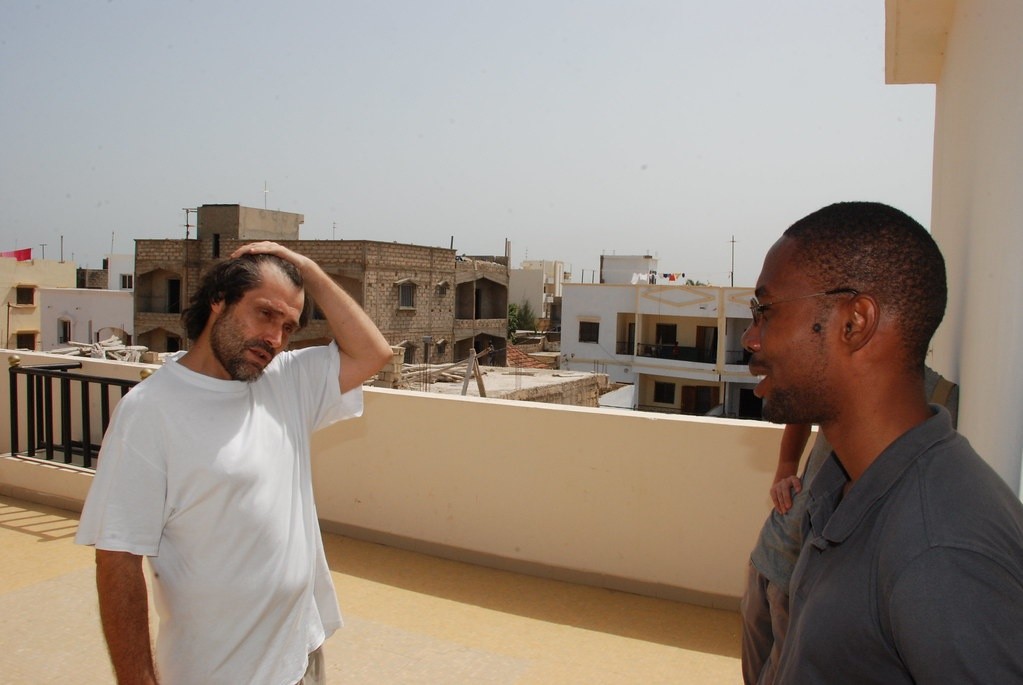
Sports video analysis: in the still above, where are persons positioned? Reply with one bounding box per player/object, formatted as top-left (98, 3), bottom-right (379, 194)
top-left (73, 241), bottom-right (393, 685)
top-left (741, 201), bottom-right (1023, 685)
top-left (770, 364), bottom-right (960, 514)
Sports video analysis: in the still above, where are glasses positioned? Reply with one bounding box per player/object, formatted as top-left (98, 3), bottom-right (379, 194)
top-left (749, 286), bottom-right (859, 327)
